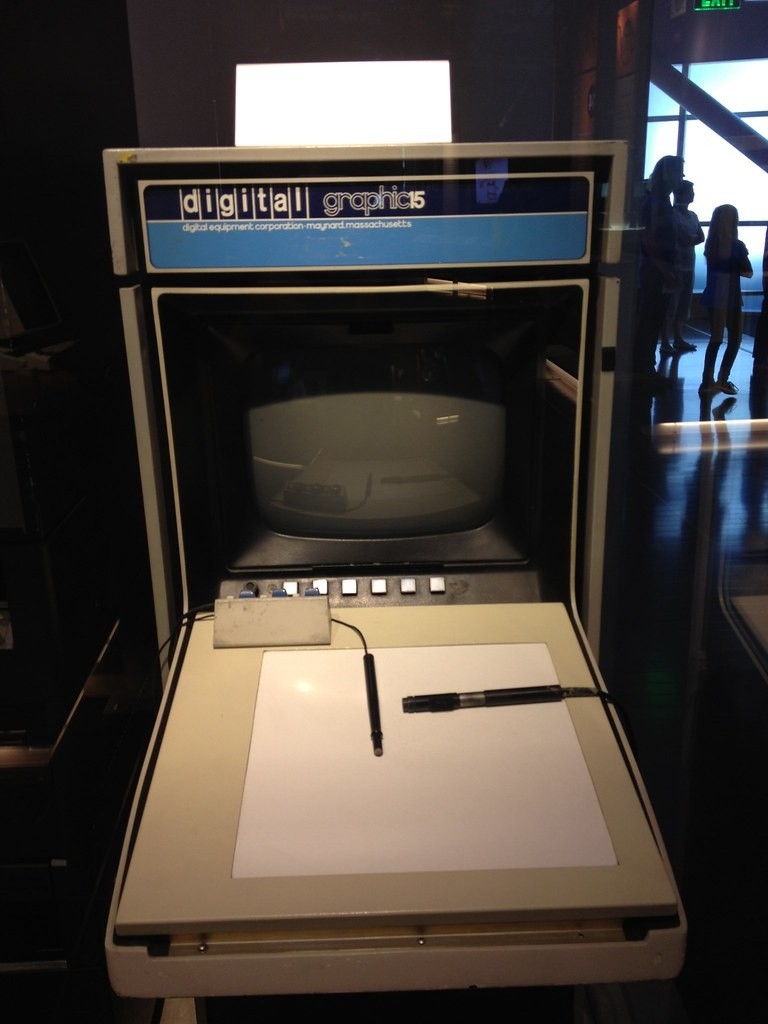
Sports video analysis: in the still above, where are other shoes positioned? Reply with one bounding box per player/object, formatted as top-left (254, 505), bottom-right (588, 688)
top-left (674, 341), bottom-right (696, 349)
top-left (699, 381), bottom-right (721, 394)
top-left (716, 380), bottom-right (737, 394)
top-left (660, 342), bottom-right (679, 353)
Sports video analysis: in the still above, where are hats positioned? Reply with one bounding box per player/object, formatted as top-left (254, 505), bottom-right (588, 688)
top-left (673, 181), bottom-right (691, 195)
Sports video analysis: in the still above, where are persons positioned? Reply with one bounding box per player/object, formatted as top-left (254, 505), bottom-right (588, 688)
top-left (634, 156), bottom-right (704, 386)
top-left (753, 229), bottom-right (768, 370)
top-left (699, 205), bottom-right (752, 394)
